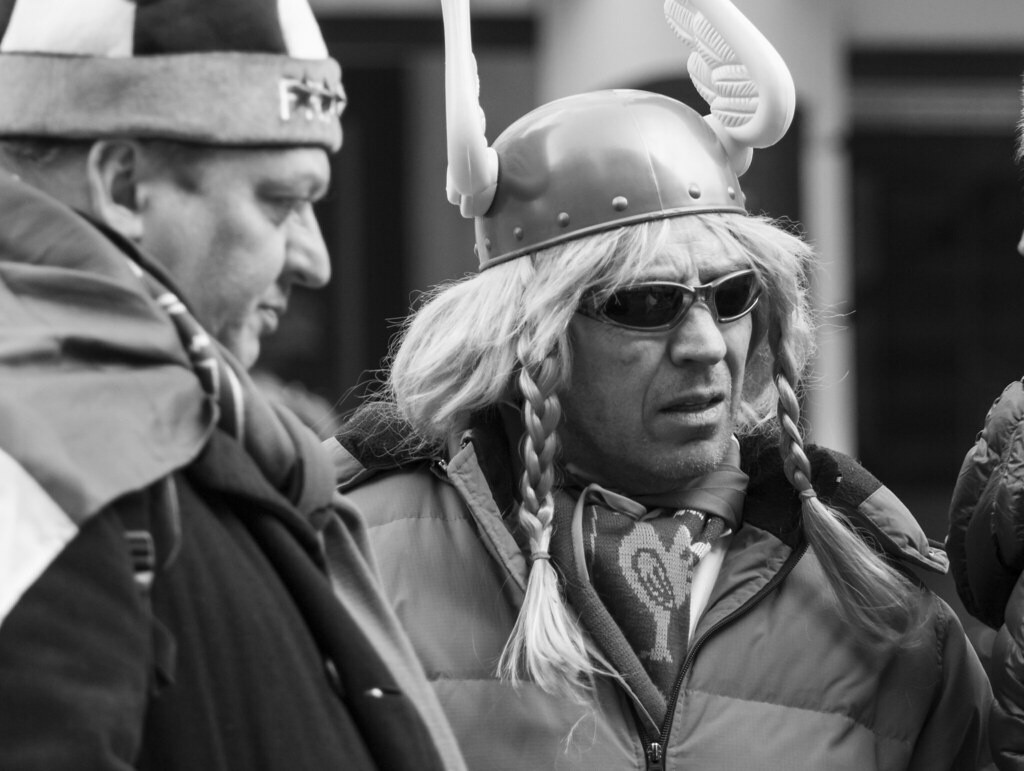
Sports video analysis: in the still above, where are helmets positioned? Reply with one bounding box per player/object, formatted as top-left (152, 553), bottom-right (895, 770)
top-left (440, 0), bottom-right (796, 272)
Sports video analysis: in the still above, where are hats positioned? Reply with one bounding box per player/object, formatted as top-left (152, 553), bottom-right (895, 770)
top-left (0, 0), bottom-right (346, 153)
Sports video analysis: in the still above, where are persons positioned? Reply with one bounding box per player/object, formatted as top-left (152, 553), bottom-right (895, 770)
top-left (0, 0), bottom-right (1024, 771)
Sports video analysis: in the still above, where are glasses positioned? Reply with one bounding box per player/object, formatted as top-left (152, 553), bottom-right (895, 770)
top-left (575, 268), bottom-right (767, 332)
